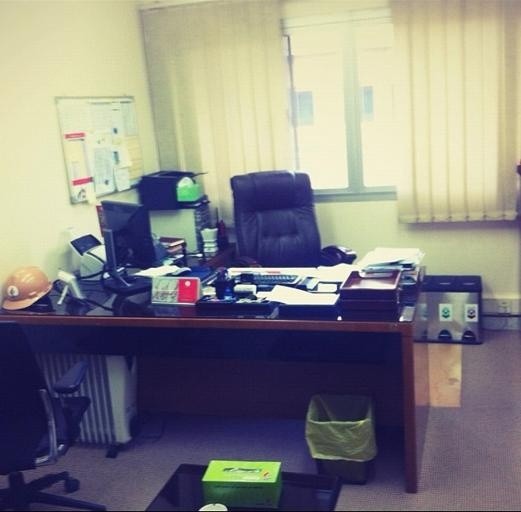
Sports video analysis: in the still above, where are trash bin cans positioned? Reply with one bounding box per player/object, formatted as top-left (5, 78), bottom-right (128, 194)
top-left (307, 393), bottom-right (374, 485)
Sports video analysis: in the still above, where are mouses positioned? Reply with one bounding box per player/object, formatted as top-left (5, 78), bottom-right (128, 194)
top-left (171, 267), bottom-right (192, 276)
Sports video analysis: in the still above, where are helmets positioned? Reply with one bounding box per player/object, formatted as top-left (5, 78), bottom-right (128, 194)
top-left (2, 266), bottom-right (52, 310)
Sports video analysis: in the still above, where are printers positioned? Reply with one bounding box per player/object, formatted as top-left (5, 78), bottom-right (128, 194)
top-left (68, 233), bottom-right (125, 281)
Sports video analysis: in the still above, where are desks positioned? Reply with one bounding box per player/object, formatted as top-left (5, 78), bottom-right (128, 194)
top-left (0, 273), bottom-right (431, 495)
top-left (144, 463), bottom-right (342, 512)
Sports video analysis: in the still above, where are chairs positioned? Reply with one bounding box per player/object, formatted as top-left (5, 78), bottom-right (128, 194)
top-left (230, 170), bottom-right (358, 266)
top-left (0, 320), bottom-right (105, 511)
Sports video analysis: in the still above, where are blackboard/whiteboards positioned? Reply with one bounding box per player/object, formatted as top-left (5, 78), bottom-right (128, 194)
top-left (54, 96), bottom-right (144, 204)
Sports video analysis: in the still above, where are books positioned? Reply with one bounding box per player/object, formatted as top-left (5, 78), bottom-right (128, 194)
top-left (159, 236), bottom-right (186, 248)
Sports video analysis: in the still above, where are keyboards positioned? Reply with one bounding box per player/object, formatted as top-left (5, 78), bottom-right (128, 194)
top-left (228, 273), bottom-right (302, 285)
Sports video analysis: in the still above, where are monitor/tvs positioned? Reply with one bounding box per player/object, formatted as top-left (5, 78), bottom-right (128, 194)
top-left (101, 200), bottom-right (159, 294)
